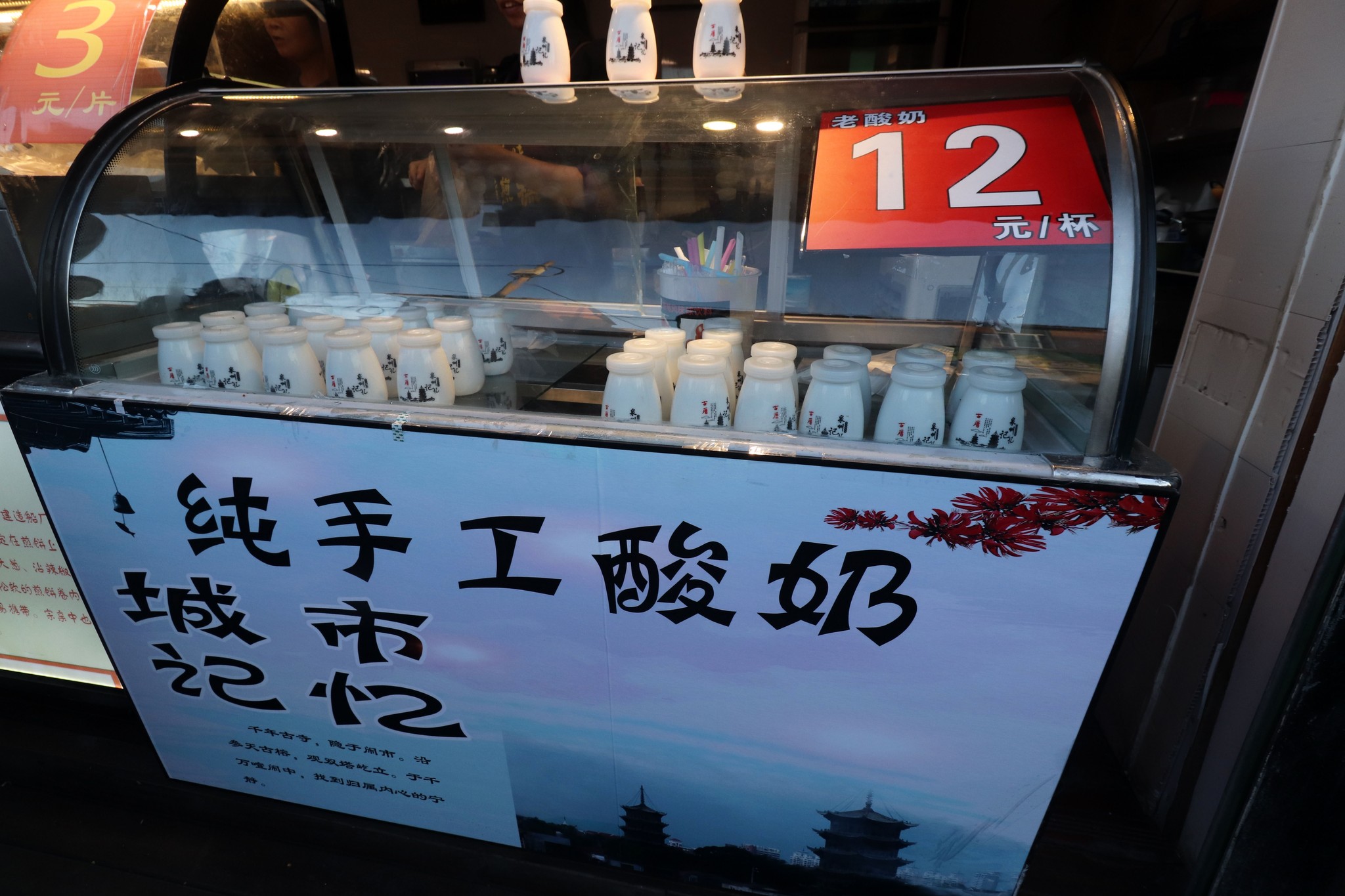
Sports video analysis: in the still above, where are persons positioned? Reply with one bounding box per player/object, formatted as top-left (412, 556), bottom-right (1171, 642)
top-left (128, 0), bottom-right (402, 218)
top-left (408, 0), bottom-right (618, 222)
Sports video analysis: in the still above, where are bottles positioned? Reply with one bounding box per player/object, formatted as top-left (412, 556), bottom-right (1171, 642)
top-left (703, 316), bottom-right (742, 329)
top-left (670, 353), bottom-right (732, 427)
top-left (947, 365), bottom-right (1028, 450)
top-left (798, 359), bottom-right (864, 440)
top-left (151, 292), bottom-right (515, 409)
top-left (873, 362), bottom-right (947, 445)
top-left (733, 355), bottom-right (797, 435)
top-left (750, 341), bottom-right (799, 412)
top-left (945, 349), bottom-right (1017, 441)
top-left (822, 344), bottom-right (872, 432)
top-left (692, 0), bottom-right (746, 100)
top-left (645, 327), bottom-right (687, 391)
top-left (519, 0), bottom-right (575, 102)
top-left (605, 0), bottom-right (660, 103)
top-left (702, 327), bottom-right (746, 406)
top-left (686, 339), bottom-right (736, 421)
top-left (600, 352), bottom-right (663, 426)
top-left (623, 337), bottom-right (674, 422)
top-left (894, 347), bottom-right (947, 368)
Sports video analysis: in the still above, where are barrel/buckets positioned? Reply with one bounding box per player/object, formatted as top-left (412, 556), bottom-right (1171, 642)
top-left (656, 265), bottom-right (762, 358)
